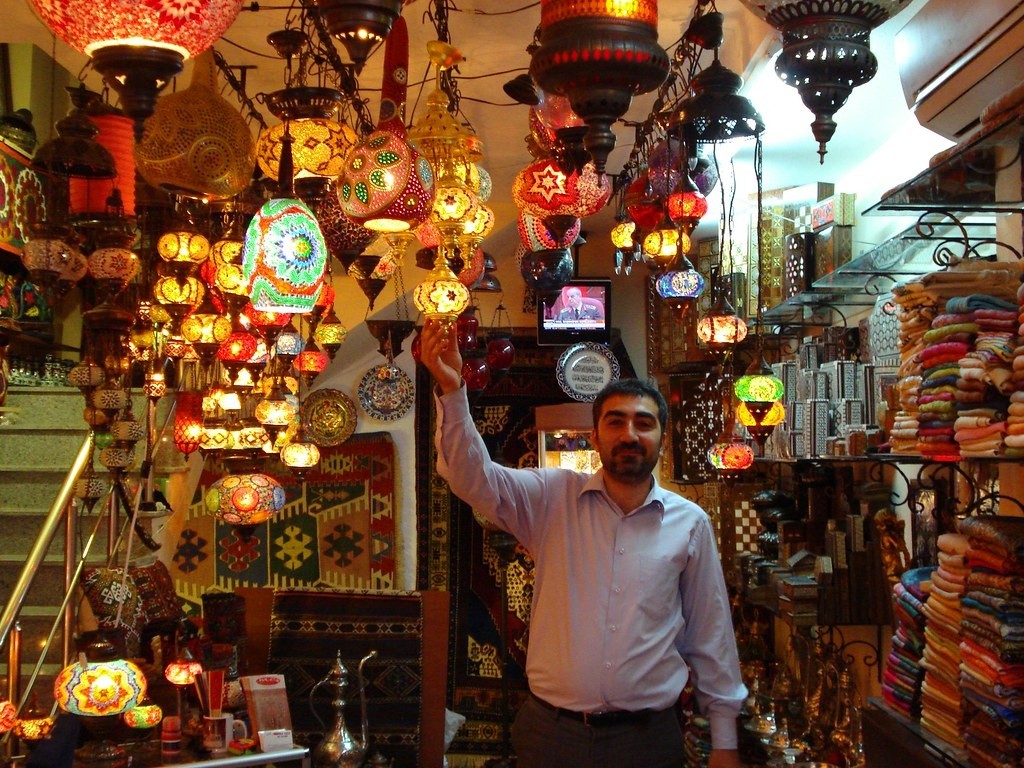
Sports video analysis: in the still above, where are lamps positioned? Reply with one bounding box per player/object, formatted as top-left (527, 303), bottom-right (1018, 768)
top-left (0, 0), bottom-right (912, 743)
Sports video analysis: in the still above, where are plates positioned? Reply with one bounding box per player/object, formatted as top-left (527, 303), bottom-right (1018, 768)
top-left (358, 365), bottom-right (414, 420)
top-left (301, 389), bottom-right (357, 447)
top-left (556, 342), bottom-right (620, 403)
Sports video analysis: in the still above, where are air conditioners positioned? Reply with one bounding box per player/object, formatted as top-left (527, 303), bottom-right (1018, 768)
top-left (893, 0), bottom-right (1024, 145)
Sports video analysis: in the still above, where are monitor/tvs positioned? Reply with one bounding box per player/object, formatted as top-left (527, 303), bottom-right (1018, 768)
top-left (536, 279), bottom-right (612, 347)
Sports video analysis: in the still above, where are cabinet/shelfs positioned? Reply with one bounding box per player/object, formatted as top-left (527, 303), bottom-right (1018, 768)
top-left (747, 201), bottom-right (1024, 768)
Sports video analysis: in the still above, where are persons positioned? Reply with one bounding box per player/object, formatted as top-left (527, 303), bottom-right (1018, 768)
top-left (557, 287), bottom-right (603, 320)
top-left (414, 316), bottom-right (751, 768)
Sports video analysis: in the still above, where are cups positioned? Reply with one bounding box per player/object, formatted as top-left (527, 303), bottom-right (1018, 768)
top-left (203, 713), bottom-right (247, 753)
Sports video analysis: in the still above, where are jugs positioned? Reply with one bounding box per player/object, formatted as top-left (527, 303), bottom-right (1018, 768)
top-left (734, 610), bottom-right (867, 768)
top-left (308, 648), bottom-right (377, 767)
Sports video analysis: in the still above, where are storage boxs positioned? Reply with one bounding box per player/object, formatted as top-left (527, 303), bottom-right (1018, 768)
top-left (771, 344), bottom-right (876, 457)
